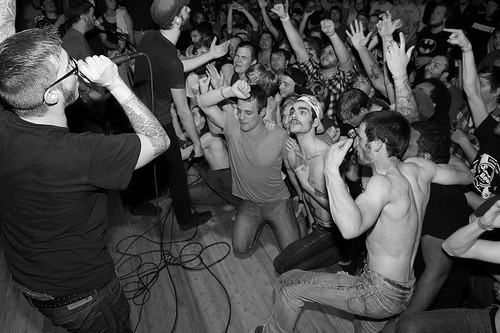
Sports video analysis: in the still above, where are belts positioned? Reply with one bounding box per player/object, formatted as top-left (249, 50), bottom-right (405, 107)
top-left (384, 278), bottom-right (410, 291)
top-left (314, 217), bottom-right (334, 227)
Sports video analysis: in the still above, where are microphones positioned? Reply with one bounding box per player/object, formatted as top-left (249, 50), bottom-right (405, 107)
top-left (80, 53), bottom-right (144, 77)
top-left (94, 20), bottom-right (118, 28)
top-left (189, 150), bottom-right (195, 161)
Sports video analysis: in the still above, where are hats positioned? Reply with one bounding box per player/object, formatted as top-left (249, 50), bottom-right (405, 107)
top-left (150, 0), bottom-right (190, 25)
top-left (69, 1), bottom-right (92, 19)
top-left (297, 94), bottom-right (325, 134)
top-left (283, 68), bottom-right (308, 86)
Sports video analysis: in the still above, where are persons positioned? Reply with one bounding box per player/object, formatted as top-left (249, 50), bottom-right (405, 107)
top-left (0, 0), bottom-right (500, 333)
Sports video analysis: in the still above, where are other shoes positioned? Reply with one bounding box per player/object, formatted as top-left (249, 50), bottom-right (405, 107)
top-left (255, 326), bottom-right (264, 333)
top-left (130, 203), bottom-right (162, 216)
top-left (179, 210), bottom-right (212, 231)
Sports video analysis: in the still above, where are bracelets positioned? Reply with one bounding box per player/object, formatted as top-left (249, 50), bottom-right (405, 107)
top-left (220, 87), bottom-right (227, 99)
top-left (462, 47), bottom-right (473, 53)
top-left (478, 216), bottom-right (491, 231)
top-left (325, 30), bottom-right (335, 36)
top-left (391, 72), bottom-right (408, 80)
top-left (279, 13), bottom-right (290, 22)
top-left (172, 114), bottom-right (178, 117)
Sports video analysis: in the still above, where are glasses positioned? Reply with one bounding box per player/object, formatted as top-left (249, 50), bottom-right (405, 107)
top-left (42, 56), bottom-right (78, 102)
